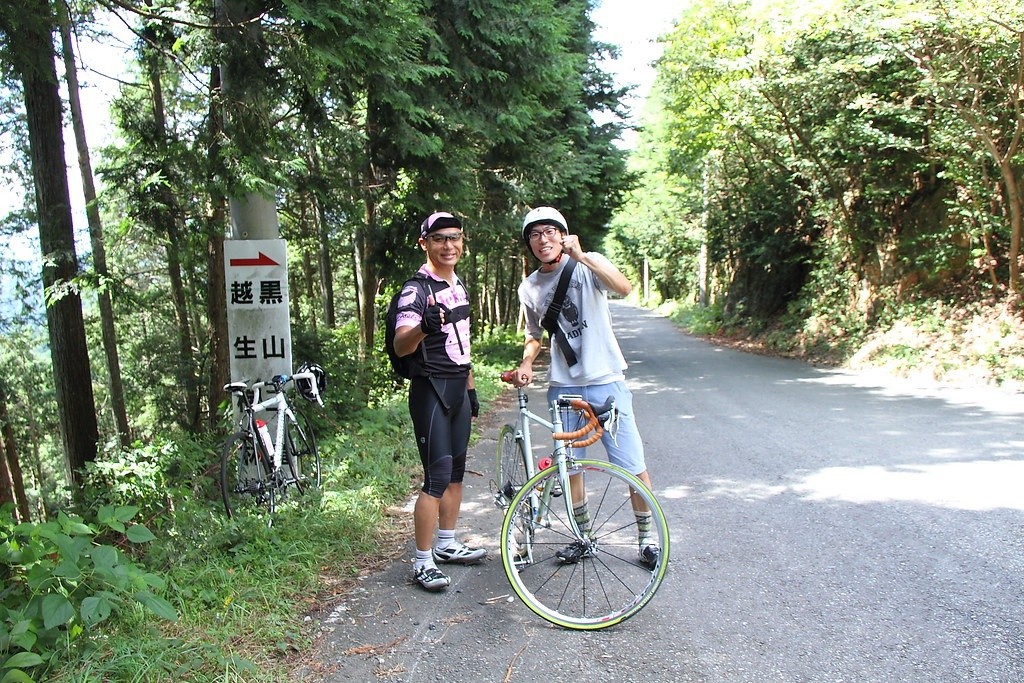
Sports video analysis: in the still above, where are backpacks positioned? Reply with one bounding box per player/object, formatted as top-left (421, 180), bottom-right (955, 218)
top-left (385, 276), bottom-right (437, 380)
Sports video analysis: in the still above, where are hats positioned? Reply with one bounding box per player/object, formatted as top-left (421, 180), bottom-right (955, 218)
top-left (420, 212), bottom-right (462, 237)
top-left (522, 206), bottom-right (569, 239)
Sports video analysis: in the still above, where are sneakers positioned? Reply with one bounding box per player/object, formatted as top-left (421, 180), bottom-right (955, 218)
top-left (638, 544), bottom-right (659, 564)
top-left (556, 537), bottom-right (599, 563)
top-left (413, 560), bottom-right (450, 589)
top-left (432, 541), bottom-right (487, 564)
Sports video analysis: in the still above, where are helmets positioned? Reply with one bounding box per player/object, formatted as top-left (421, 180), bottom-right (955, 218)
top-left (296, 363), bottom-right (326, 402)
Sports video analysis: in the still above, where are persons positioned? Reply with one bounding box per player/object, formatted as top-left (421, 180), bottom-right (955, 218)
top-left (515, 207), bottom-right (672, 571)
top-left (394, 210), bottom-right (490, 587)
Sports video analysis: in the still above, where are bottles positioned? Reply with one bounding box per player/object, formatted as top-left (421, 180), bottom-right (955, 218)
top-left (255, 419), bottom-right (275, 456)
top-left (534, 458), bottom-right (553, 492)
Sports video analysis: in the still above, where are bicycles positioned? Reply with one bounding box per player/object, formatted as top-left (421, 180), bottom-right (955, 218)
top-left (490, 367), bottom-right (671, 632)
top-left (216, 365), bottom-right (323, 530)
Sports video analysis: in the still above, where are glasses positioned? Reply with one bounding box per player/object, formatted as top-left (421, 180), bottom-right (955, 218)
top-left (528, 227), bottom-right (560, 241)
top-left (425, 234), bottom-right (462, 242)
